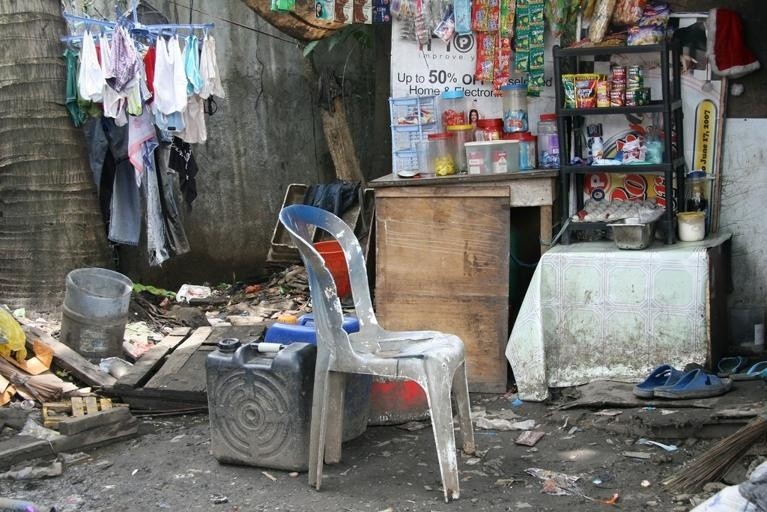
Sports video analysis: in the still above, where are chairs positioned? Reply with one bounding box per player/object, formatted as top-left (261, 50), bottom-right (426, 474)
top-left (278, 205), bottom-right (475, 504)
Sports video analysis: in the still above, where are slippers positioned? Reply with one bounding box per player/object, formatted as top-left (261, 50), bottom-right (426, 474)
top-left (654, 369), bottom-right (733, 398)
top-left (717, 357), bottom-right (747, 377)
top-left (634, 365), bottom-right (686, 398)
top-left (729, 361), bottom-right (767, 381)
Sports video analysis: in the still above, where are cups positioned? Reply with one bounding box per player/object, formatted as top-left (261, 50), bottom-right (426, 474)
top-left (635, 87), bottom-right (651, 106)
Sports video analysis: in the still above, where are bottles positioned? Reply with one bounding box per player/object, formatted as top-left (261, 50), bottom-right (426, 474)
top-left (429, 133), bottom-right (457, 177)
top-left (447, 125), bottom-right (474, 175)
top-left (502, 84), bottom-right (529, 133)
top-left (538, 113), bottom-right (560, 169)
top-left (474, 119), bottom-right (503, 141)
top-left (440, 91), bottom-right (467, 133)
top-left (626, 65), bottom-right (643, 88)
top-left (687, 172), bottom-right (707, 211)
top-left (677, 213), bottom-right (705, 242)
top-left (519, 136), bottom-right (538, 170)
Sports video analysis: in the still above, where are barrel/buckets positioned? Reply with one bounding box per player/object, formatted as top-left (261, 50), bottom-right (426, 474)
top-left (204, 338), bottom-right (317, 473)
top-left (60, 267), bottom-right (135, 365)
top-left (263, 314), bottom-right (369, 442)
top-left (313, 240), bottom-right (349, 297)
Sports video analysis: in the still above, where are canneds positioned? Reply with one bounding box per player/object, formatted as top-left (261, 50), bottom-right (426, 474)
top-left (643, 88), bottom-right (651, 105)
top-left (595, 80), bottom-right (609, 108)
top-left (626, 89), bottom-right (643, 106)
top-left (610, 89), bottom-right (626, 107)
top-left (613, 65), bottom-right (626, 89)
top-left (591, 137), bottom-right (603, 161)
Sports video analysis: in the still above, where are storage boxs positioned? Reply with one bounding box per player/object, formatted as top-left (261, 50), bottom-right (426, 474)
top-left (464, 138), bottom-right (521, 177)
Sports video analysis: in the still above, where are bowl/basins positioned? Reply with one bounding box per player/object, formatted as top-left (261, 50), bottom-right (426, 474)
top-left (606, 218), bottom-right (655, 250)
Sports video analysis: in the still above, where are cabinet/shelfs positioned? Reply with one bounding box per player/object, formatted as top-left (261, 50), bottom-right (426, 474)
top-left (552, 30), bottom-right (685, 246)
top-left (368, 169), bottom-right (563, 394)
top-left (542, 233), bottom-right (734, 386)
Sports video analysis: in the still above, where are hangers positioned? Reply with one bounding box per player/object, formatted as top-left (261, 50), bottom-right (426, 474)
top-left (57, 0), bottom-right (216, 51)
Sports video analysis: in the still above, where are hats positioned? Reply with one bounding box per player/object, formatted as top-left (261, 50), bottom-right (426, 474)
top-left (676, 22), bottom-right (706, 52)
top-left (701, 8), bottom-right (760, 96)
